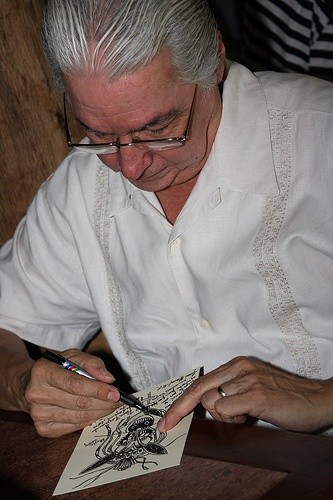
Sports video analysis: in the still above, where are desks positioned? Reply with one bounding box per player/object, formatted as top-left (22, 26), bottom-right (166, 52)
top-left (0, 410), bottom-right (333, 499)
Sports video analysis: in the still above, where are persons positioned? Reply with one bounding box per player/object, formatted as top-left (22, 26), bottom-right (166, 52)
top-left (0, 0), bottom-right (333, 437)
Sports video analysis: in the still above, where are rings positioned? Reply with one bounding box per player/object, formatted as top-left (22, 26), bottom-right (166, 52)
top-left (215, 385), bottom-right (226, 398)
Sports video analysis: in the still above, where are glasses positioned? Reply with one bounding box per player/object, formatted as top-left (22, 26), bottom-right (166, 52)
top-left (61, 82), bottom-right (198, 155)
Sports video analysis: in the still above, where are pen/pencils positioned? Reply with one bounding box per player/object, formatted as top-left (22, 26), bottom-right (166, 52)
top-left (42, 349), bottom-right (149, 413)
